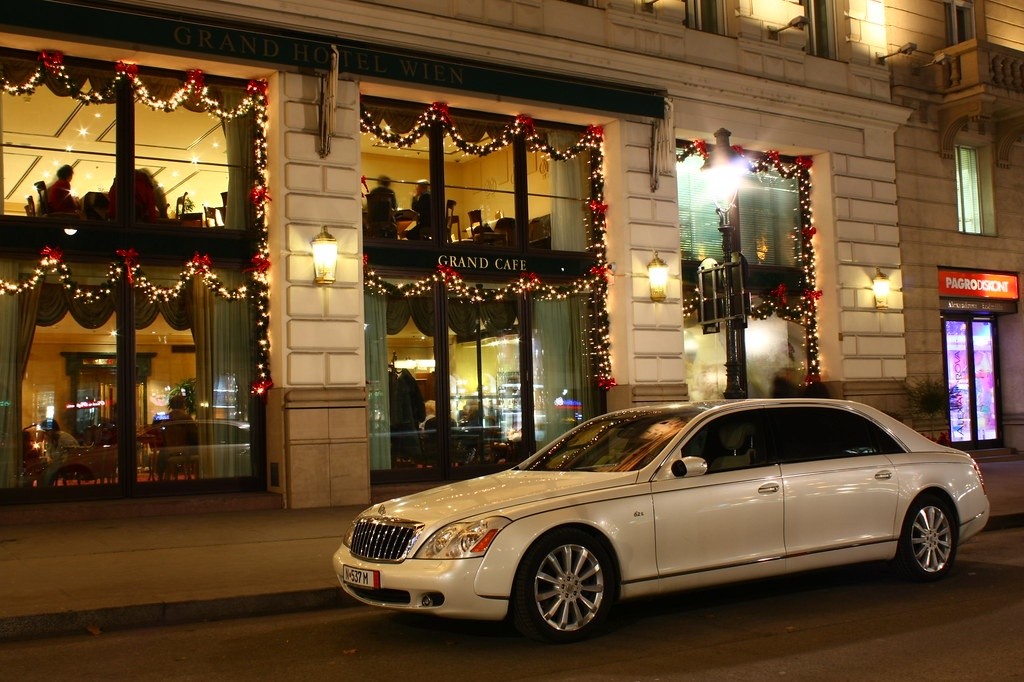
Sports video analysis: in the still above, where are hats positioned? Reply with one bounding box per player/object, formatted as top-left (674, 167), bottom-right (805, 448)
top-left (771, 356), bottom-right (795, 369)
top-left (415, 178), bottom-right (429, 185)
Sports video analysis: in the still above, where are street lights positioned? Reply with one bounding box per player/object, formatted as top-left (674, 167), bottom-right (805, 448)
top-left (699, 128), bottom-right (749, 397)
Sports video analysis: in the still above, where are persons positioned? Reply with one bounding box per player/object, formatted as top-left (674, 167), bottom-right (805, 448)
top-left (368, 176), bottom-right (398, 240)
top-left (407, 179), bottom-right (431, 240)
top-left (47, 165), bottom-right (86, 219)
top-left (169, 396), bottom-right (193, 419)
top-left (40, 419), bottom-right (79, 488)
top-left (140, 169), bottom-right (167, 218)
top-left (420, 400), bottom-right (504, 429)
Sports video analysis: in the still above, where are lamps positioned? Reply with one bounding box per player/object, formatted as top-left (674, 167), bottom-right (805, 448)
top-left (766, 16), bottom-right (808, 42)
top-left (646, 250), bottom-right (669, 301)
top-left (871, 268), bottom-right (891, 311)
top-left (311, 226), bottom-right (336, 287)
top-left (875, 41), bottom-right (917, 65)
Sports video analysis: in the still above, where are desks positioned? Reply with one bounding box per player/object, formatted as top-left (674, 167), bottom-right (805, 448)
top-left (363, 208), bottom-right (419, 239)
top-left (461, 217), bottom-right (540, 245)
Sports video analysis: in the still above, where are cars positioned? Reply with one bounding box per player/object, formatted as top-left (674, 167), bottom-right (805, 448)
top-left (331, 398), bottom-right (990, 643)
top-left (40, 420), bottom-right (254, 488)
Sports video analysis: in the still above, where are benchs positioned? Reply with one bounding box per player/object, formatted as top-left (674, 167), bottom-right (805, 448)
top-left (529, 213), bottom-right (550, 247)
top-left (370, 426), bottom-right (501, 468)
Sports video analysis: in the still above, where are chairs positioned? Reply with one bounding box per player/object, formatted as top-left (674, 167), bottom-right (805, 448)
top-left (175, 192), bottom-right (188, 219)
top-left (711, 420), bottom-right (760, 468)
top-left (203, 204), bottom-right (219, 227)
top-left (447, 215), bottom-right (473, 243)
top-left (468, 209), bottom-right (505, 246)
top-left (421, 200), bottom-right (457, 241)
top-left (364, 193), bottom-right (398, 240)
top-left (24, 179), bottom-right (77, 219)
